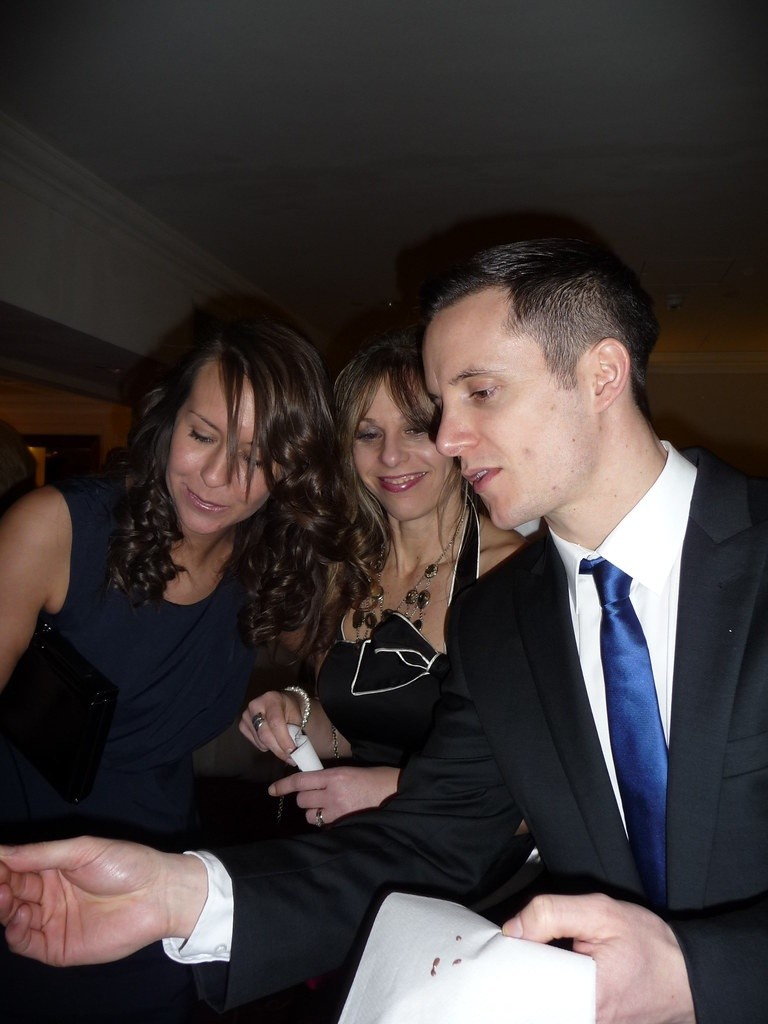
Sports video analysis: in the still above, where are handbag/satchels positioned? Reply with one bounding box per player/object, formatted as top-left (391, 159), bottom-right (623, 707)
top-left (1, 607), bottom-right (122, 808)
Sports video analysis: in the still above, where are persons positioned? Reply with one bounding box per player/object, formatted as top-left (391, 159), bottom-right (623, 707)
top-left (238, 328), bottom-right (536, 1023)
top-left (0, 209), bottom-right (768, 1024)
top-left (0, 292), bottom-right (353, 1024)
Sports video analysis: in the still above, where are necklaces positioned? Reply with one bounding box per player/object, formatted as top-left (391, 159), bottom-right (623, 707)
top-left (347, 502), bottom-right (466, 641)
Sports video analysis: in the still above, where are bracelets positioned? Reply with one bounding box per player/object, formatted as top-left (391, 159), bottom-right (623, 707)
top-left (282, 687), bottom-right (312, 729)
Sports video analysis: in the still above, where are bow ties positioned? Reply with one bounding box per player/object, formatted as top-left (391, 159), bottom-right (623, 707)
top-left (584, 550), bottom-right (671, 905)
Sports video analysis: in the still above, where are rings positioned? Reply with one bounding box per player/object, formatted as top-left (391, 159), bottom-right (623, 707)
top-left (249, 713), bottom-right (265, 731)
top-left (315, 807), bottom-right (325, 828)
